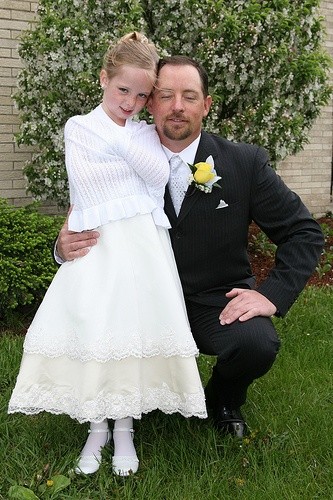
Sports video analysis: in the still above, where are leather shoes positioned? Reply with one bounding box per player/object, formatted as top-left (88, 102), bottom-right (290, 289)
top-left (208, 405), bottom-right (250, 438)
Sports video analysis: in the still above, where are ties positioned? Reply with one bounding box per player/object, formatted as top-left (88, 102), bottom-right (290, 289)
top-left (168, 155), bottom-right (187, 217)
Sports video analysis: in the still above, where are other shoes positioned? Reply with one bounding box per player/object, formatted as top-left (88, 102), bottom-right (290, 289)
top-left (112, 428), bottom-right (140, 476)
top-left (75, 429), bottom-right (111, 474)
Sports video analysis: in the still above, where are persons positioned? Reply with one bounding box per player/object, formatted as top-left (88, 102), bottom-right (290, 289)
top-left (8, 31), bottom-right (208, 477)
top-left (50, 56), bottom-right (325, 449)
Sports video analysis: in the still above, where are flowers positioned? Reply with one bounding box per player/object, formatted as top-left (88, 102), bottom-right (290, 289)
top-left (188, 155), bottom-right (222, 194)
